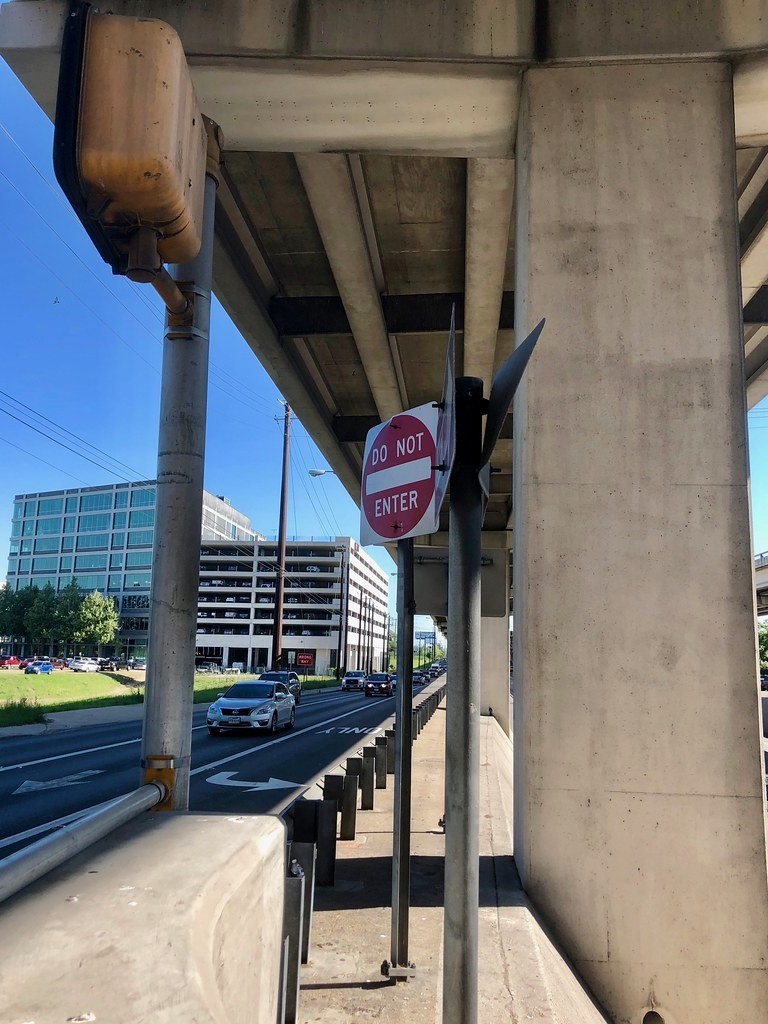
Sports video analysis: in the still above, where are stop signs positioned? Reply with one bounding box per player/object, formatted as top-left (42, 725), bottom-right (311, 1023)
top-left (360, 400), bottom-right (438, 546)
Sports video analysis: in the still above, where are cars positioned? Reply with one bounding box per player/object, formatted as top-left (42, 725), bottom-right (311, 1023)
top-left (257, 629), bottom-right (268, 635)
top-left (307, 565), bottom-right (320, 572)
top-left (225, 612), bottom-right (318, 619)
top-left (203, 549), bottom-right (209, 555)
top-left (286, 630), bottom-right (298, 636)
top-left (198, 612), bottom-right (207, 617)
top-left (224, 629), bottom-right (232, 634)
top-left (228, 566), bottom-right (236, 570)
top-left (240, 628), bottom-right (249, 634)
top-left (207, 680), bottom-right (296, 737)
top-left (197, 628), bottom-right (207, 634)
top-left (200, 579), bottom-right (316, 587)
top-left (391, 659), bottom-right (447, 689)
top-left (302, 630), bottom-right (314, 636)
top-left (198, 596), bottom-right (298, 603)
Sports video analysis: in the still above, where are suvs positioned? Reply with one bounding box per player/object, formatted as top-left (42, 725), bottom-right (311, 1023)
top-left (197, 662), bottom-right (226, 674)
top-left (365, 674), bottom-right (393, 697)
top-left (0, 656), bottom-right (146, 675)
top-left (258, 669), bottom-right (302, 704)
top-left (342, 670), bottom-right (368, 691)
